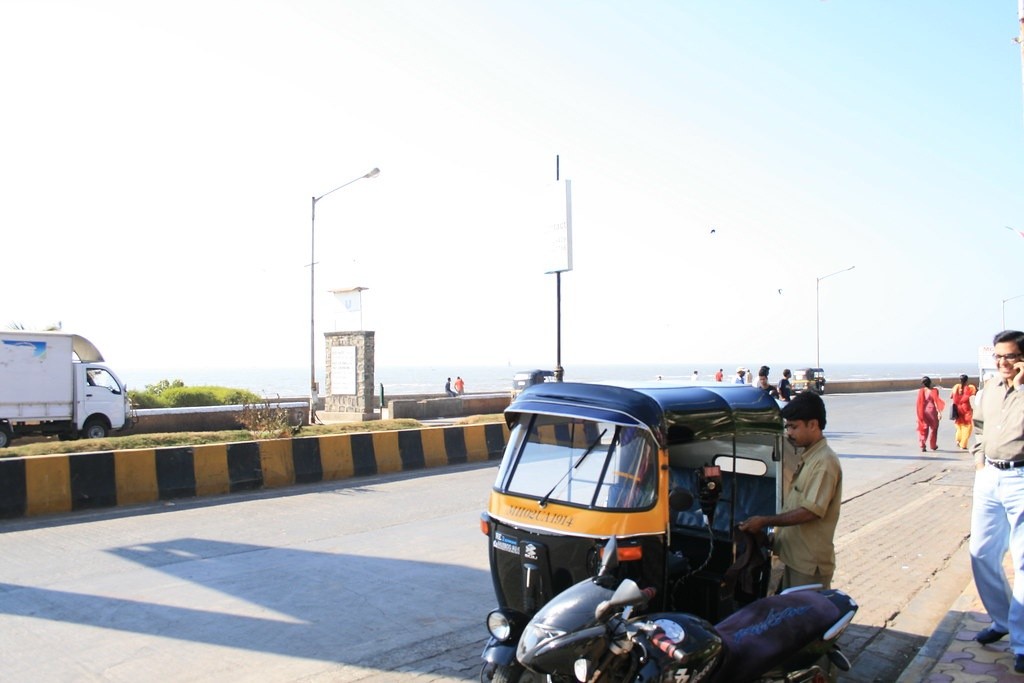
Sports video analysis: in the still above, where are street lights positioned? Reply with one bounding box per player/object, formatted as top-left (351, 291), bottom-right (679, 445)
top-left (310, 167), bottom-right (381, 424)
top-left (816, 266), bottom-right (856, 377)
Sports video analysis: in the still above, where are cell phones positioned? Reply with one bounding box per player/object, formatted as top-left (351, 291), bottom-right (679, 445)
top-left (1016, 359), bottom-right (1024, 374)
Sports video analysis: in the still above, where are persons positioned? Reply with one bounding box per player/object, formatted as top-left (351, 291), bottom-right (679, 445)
top-left (970, 330), bottom-right (1024, 673)
top-left (917, 376), bottom-right (938, 452)
top-left (735, 368), bottom-right (753, 384)
top-left (454, 377), bottom-right (464, 395)
top-left (691, 371), bottom-right (699, 381)
top-left (445, 378), bottom-right (455, 398)
top-left (739, 391), bottom-right (842, 591)
top-left (586, 425), bottom-right (709, 614)
top-left (657, 376), bottom-right (661, 381)
top-left (752, 366), bottom-right (779, 400)
top-left (777, 369), bottom-right (791, 401)
top-left (950, 375), bottom-right (972, 450)
top-left (716, 368), bottom-right (723, 382)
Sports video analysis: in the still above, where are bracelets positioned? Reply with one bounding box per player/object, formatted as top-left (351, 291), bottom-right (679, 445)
top-left (1014, 384), bottom-right (1024, 392)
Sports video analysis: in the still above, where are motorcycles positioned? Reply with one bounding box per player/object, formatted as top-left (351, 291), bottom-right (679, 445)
top-left (515, 533), bottom-right (858, 683)
top-left (792, 368), bottom-right (826, 396)
top-left (480, 382), bottom-right (809, 683)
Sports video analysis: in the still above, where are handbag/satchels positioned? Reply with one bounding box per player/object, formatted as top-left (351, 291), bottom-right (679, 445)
top-left (948, 403), bottom-right (958, 420)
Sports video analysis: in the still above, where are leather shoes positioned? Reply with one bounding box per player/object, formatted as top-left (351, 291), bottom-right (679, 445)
top-left (1013, 654), bottom-right (1024, 673)
top-left (976, 627), bottom-right (1008, 644)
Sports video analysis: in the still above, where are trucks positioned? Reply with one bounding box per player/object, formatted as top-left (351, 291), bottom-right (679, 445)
top-left (0, 331), bottom-right (142, 448)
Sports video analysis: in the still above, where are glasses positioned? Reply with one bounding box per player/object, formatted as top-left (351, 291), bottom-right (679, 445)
top-left (992, 353), bottom-right (1023, 359)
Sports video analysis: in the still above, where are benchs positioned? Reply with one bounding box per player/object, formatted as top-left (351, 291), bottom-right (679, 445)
top-left (607, 464), bottom-right (776, 542)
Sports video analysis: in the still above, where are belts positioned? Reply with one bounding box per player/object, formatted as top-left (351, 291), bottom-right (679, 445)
top-left (986, 459), bottom-right (1024, 471)
